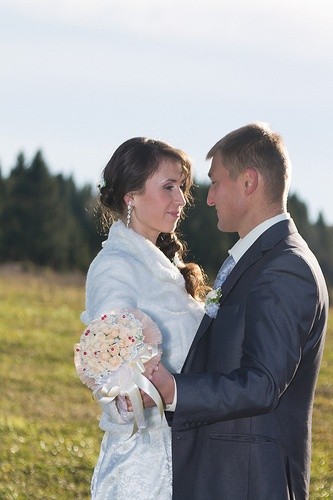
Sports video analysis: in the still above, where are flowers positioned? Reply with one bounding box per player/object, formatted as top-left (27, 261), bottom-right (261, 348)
top-left (204, 287), bottom-right (223, 319)
top-left (72, 304), bottom-right (163, 443)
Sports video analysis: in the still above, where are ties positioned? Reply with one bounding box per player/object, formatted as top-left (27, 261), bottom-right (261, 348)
top-left (213, 252), bottom-right (235, 294)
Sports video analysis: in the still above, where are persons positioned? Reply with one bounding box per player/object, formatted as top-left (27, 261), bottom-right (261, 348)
top-left (124, 125), bottom-right (329, 500)
top-left (80, 136), bottom-right (213, 500)
